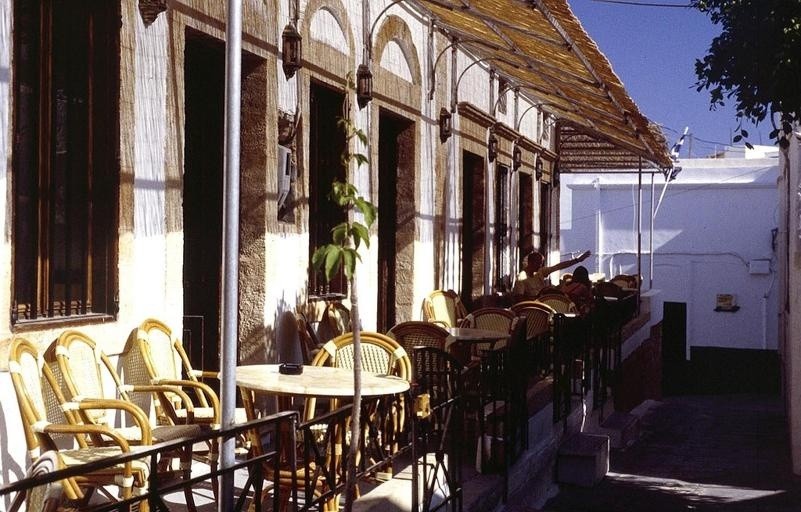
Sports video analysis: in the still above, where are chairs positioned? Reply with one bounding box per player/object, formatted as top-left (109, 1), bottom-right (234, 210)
top-left (9, 337), bottom-right (163, 512)
top-left (462, 306), bottom-right (516, 385)
top-left (385, 321), bottom-right (460, 421)
top-left (609, 274), bottom-right (637, 298)
top-left (592, 282), bottom-right (627, 320)
top-left (422, 290), bottom-right (464, 337)
top-left (135, 317), bottom-right (265, 511)
top-left (301, 331), bottom-right (412, 486)
top-left (534, 295), bottom-right (577, 325)
top-left (510, 302), bottom-right (562, 341)
top-left (55, 329), bottom-right (211, 510)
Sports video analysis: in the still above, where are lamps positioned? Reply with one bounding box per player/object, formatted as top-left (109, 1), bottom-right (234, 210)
top-left (552, 161), bottom-right (560, 187)
top-left (488, 132), bottom-right (499, 162)
top-left (439, 107), bottom-right (453, 143)
top-left (535, 155), bottom-right (544, 181)
top-left (281, 21), bottom-right (303, 80)
top-left (356, 63), bottom-right (373, 109)
top-left (512, 144), bottom-right (522, 172)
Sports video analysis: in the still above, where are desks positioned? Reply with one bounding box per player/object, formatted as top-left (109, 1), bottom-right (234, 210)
top-left (217, 363), bottom-right (409, 510)
top-left (438, 326), bottom-right (512, 417)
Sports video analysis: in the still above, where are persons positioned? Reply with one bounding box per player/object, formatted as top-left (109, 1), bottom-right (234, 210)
top-left (562, 267), bottom-right (591, 295)
top-left (514, 250), bottom-right (591, 297)
